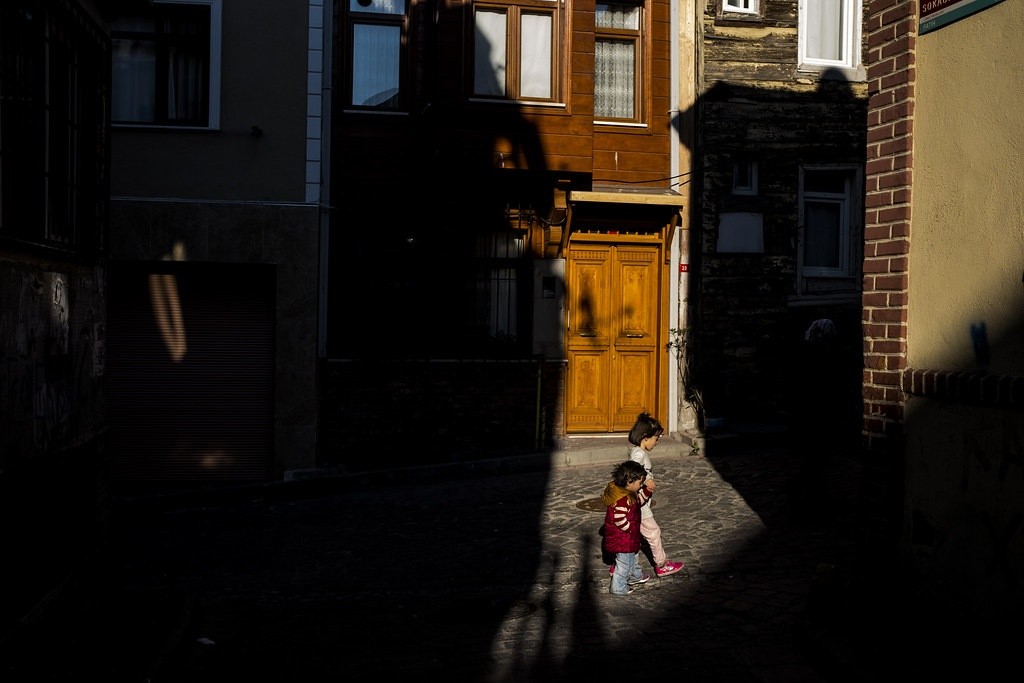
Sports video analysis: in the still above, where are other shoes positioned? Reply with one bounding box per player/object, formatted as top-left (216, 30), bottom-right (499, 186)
top-left (627, 573), bottom-right (651, 585)
top-left (609, 587), bottom-right (634, 595)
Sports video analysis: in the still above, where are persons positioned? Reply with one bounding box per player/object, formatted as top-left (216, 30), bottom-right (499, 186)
top-left (609, 413), bottom-right (683, 577)
top-left (602, 461), bottom-right (655, 595)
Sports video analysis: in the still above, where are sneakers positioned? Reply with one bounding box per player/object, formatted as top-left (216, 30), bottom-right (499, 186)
top-left (655, 560), bottom-right (685, 577)
top-left (609, 564), bottom-right (615, 576)
